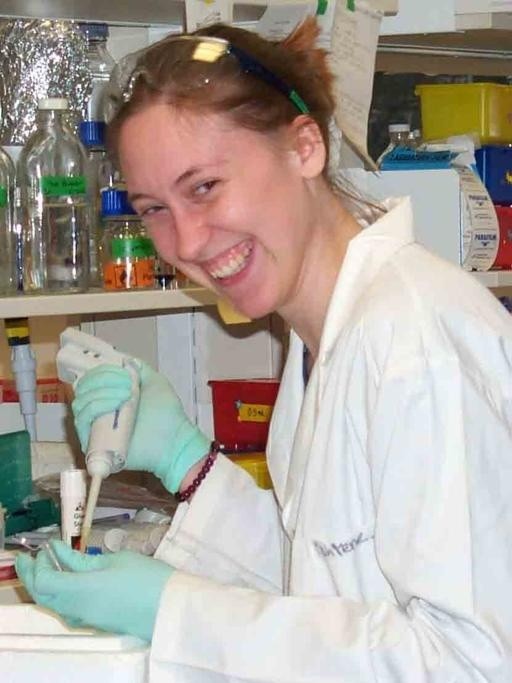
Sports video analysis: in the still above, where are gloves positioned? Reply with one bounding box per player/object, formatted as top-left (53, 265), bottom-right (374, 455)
top-left (71, 361), bottom-right (212, 495)
top-left (15, 539), bottom-right (176, 642)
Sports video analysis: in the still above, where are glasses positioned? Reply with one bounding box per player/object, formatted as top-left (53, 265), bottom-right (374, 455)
top-left (103, 35), bottom-right (311, 125)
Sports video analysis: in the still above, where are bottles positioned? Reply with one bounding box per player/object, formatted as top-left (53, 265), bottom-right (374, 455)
top-left (58, 467), bottom-right (88, 549)
top-left (377, 124), bottom-right (411, 164)
top-left (0, 22), bottom-right (176, 294)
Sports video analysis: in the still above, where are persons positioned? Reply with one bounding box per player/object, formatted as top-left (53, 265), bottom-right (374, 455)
top-left (13, 12), bottom-right (512, 683)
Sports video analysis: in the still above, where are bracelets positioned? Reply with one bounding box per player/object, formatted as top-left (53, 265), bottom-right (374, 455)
top-left (176, 439), bottom-right (220, 501)
